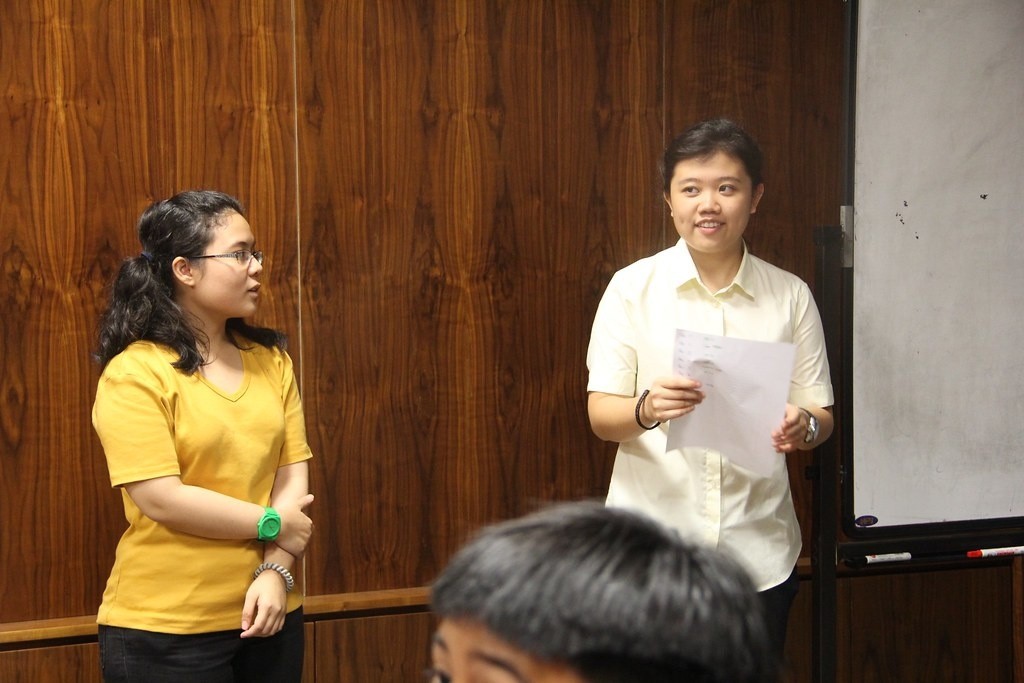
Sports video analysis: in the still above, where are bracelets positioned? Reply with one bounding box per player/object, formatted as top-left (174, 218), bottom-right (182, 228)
top-left (635, 389), bottom-right (661, 430)
top-left (253, 562), bottom-right (295, 592)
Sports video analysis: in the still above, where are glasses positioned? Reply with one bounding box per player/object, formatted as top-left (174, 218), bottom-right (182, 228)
top-left (181, 250), bottom-right (263, 266)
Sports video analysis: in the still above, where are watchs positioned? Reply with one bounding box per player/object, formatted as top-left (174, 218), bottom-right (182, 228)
top-left (256, 507), bottom-right (282, 544)
top-left (801, 408), bottom-right (820, 444)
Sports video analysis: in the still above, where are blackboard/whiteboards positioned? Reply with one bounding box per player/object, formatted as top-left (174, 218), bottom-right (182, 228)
top-left (844, 0), bottom-right (1024, 538)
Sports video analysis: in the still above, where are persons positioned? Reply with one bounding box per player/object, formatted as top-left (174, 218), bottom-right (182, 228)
top-left (91, 191), bottom-right (314, 683)
top-left (421, 501), bottom-right (774, 683)
top-left (584, 117), bottom-right (834, 660)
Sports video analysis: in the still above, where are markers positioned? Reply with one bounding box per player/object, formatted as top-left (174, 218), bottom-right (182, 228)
top-left (845, 552), bottom-right (911, 567)
top-left (967, 545), bottom-right (1024, 558)
